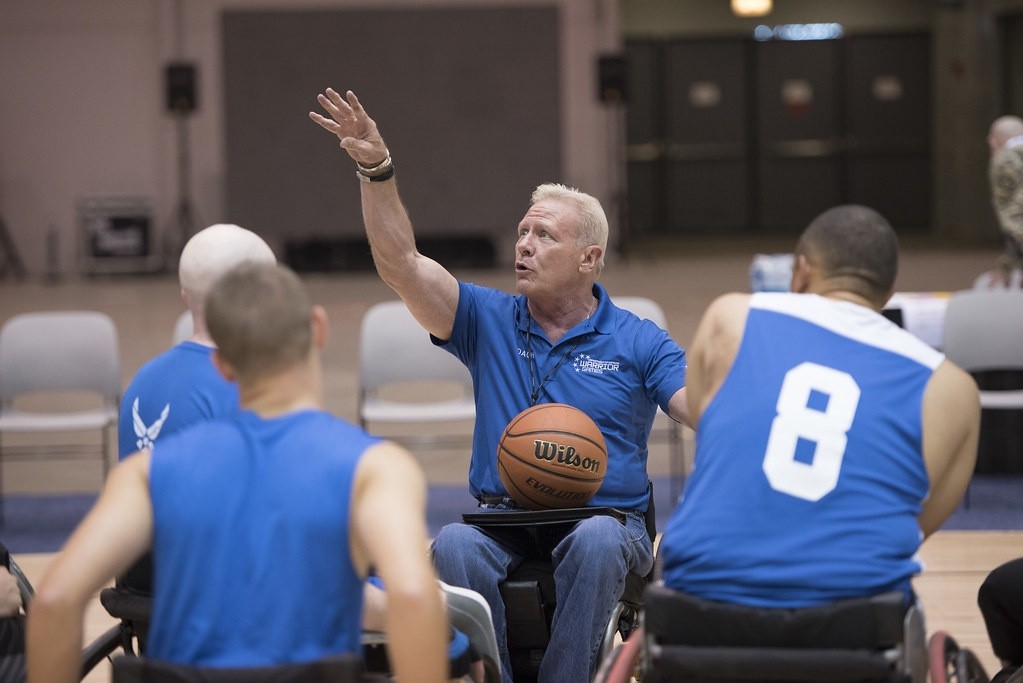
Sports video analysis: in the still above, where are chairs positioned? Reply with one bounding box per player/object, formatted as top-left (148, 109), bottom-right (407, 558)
top-left (607, 296), bottom-right (682, 501)
top-left (1, 312), bottom-right (124, 478)
top-left (940, 290), bottom-right (1023, 501)
top-left (355, 301), bottom-right (475, 435)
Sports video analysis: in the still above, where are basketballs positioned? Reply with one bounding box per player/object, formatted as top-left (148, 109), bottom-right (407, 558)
top-left (496, 401), bottom-right (609, 511)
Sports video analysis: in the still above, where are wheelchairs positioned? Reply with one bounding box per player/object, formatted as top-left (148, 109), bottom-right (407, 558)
top-left (64, 529), bottom-right (990, 683)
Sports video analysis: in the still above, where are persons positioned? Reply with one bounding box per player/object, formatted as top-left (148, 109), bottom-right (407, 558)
top-left (119, 225), bottom-right (485, 682)
top-left (977, 557), bottom-right (1023, 667)
top-left (974, 117), bottom-right (1022, 289)
top-left (0, 565), bottom-right (27, 683)
top-left (661, 207), bottom-right (980, 669)
top-left (310, 87), bottom-right (695, 683)
top-left (27, 261), bottom-right (448, 683)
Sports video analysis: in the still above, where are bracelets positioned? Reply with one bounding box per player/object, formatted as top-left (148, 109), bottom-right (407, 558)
top-left (356, 149), bottom-right (395, 182)
